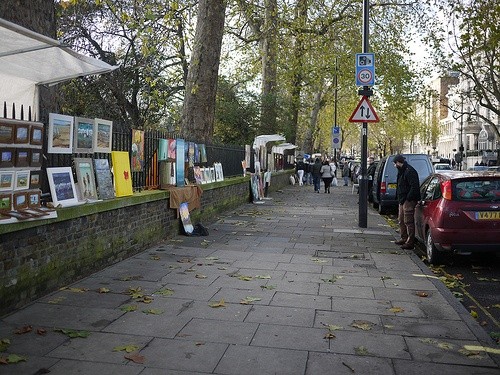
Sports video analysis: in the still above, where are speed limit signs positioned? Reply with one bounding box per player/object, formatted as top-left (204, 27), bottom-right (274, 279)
top-left (358, 69), bottom-right (372, 84)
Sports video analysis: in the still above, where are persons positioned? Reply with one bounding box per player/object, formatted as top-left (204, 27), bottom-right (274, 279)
top-left (393, 152), bottom-right (421, 250)
top-left (311, 157), bottom-right (322, 192)
top-left (451, 158), bottom-right (455, 168)
top-left (320, 160), bottom-right (333, 193)
top-left (294, 156), bottom-right (338, 186)
top-left (474, 159), bottom-right (494, 166)
top-left (366, 156), bottom-right (376, 204)
top-left (342, 161), bottom-right (349, 185)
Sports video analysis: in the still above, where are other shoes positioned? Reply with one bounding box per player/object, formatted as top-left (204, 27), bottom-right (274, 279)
top-left (343, 184), bottom-right (347, 186)
top-left (400, 244), bottom-right (414, 249)
top-left (318, 189), bottom-right (319, 193)
top-left (395, 239), bottom-right (407, 244)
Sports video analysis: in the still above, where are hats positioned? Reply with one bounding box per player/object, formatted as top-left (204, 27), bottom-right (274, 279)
top-left (316, 158), bottom-right (319, 161)
top-left (391, 154), bottom-right (405, 162)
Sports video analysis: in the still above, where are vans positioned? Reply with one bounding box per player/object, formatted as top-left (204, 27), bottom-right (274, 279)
top-left (371, 153), bottom-right (434, 215)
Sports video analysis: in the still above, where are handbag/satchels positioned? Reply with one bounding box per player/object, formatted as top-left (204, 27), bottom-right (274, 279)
top-left (331, 177), bottom-right (337, 185)
top-left (330, 166), bottom-right (335, 176)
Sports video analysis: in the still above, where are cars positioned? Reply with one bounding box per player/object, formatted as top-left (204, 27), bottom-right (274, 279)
top-left (412, 169), bottom-right (500, 265)
top-left (339, 156), bottom-right (378, 203)
top-left (434, 163), bottom-right (452, 175)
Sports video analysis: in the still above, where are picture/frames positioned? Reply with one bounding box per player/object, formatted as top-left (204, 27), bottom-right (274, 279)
top-left (47, 113), bottom-right (73, 154)
top-left (200, 168), bottom-right (216, 184)
top-left (28, 191), bottom-right (41, 207)
top-left (46, 167), bottom-right (78, 208)
top-left (0, 171), bottom-right (15, 191)
top-left (14, 170), bottom-right (30, 190)
top-left (0, 147), bottom-right (16, 168)
top-left (94, 118), bottom-right (113, 153)
top-left (0, 210), bottom-right (34, 219)
top-left (0, 122), bottom-right (15, 144)
top-left (31, 125), bottom-right (44, 145)
top-left (29, 171), bottom-right (43, 189)
top-left (0, 194), bottom-right (12, 219)
top-left (14, 124), bottom-right (30, 144)
top-left (30, 148), bottom-right (43, 167)
top-left (15, 148), bottom-right (31, 167)
top-left (73, 117), bottom-right (95, 153)
top-left (17, 208), bottom-right (50, 217)
top-left (74, 158), bottom-right (99, 201)
top-left (13, 192), bottom-right (28, 210)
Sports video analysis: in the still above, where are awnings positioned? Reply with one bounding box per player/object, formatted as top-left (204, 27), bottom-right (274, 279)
top-left (0, 17), bottom-right (120, 123)
